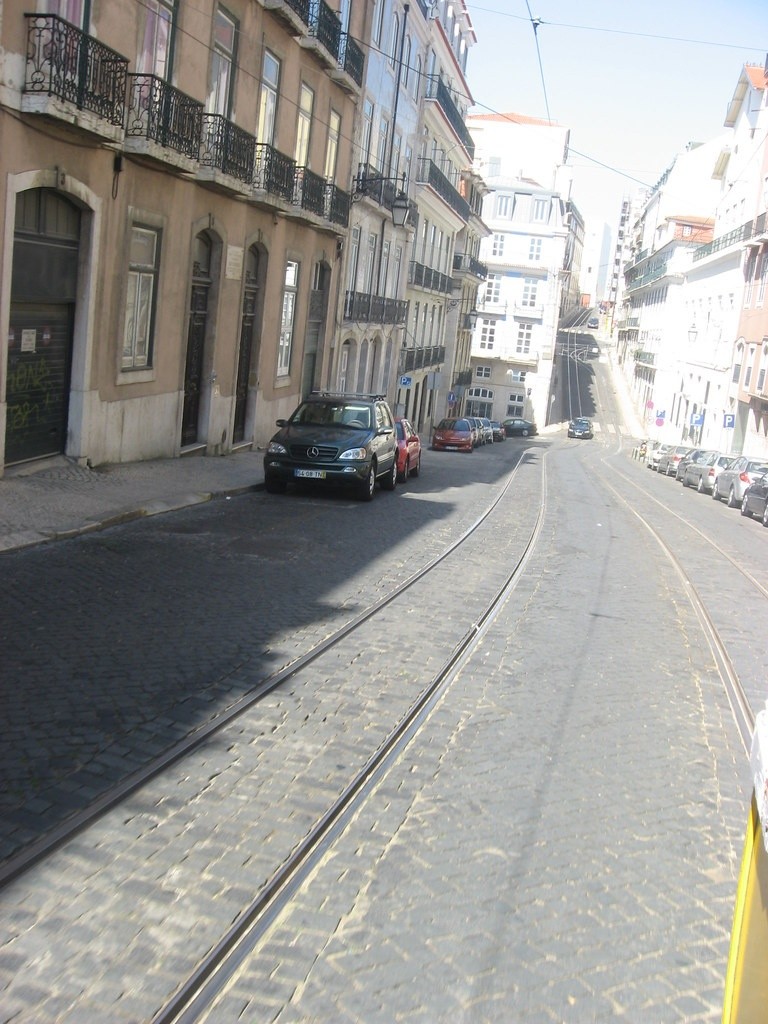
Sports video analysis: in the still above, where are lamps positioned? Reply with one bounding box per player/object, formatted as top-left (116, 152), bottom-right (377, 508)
top-left (446, 299), bottom-right (479, 328)
top-left (349, 172), bottom-right (410, 227)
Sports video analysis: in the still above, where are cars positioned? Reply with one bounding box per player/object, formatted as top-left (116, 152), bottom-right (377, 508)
top-left (675, 449), bottom-right (705, 481)
top-left (568, 418), bottom-right (593, 439)
top-left (741, 472), bottom-right (768, 527)
top-left (478, 417), bottom-right (494, 444)
top-left (683, 451), bottom-right (740, 494)
top-left (712, 456), bottom-right (768, 508)
top-left (489, 420), bottom-right (506, 442)
top-left (432, 418), bottom-right (476, 453)
top-left (473, 418), bottom-right (486, 446)
top-left (646, 443), bottom-right (674, 471)
top-left (588, 318), bottom-right (599, 329)
top-left (656, 445), bottom-right (694, 477)
top-left (393, 417), bottom-right (422, 483)
top-left (464, 417), bottom-right (479, 448)
top-left (503, 419), bottom-right (539, 437)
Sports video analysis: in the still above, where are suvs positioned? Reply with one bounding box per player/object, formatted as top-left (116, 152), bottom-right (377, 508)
top-left (264, 391), bottom-right (400, 503)
